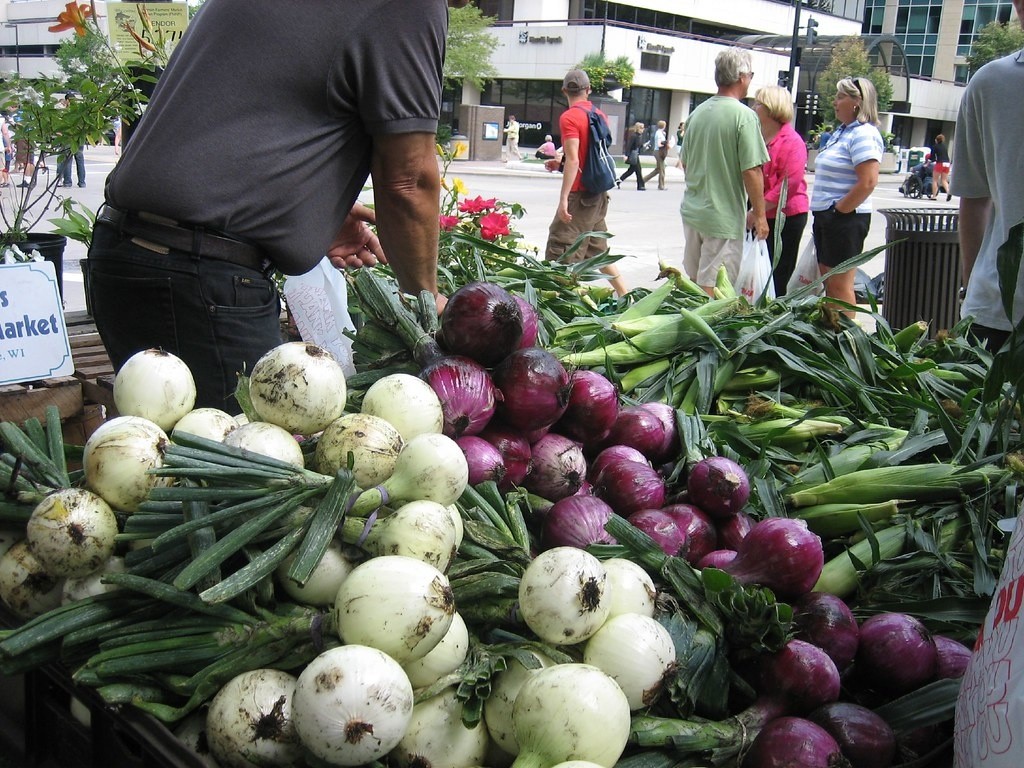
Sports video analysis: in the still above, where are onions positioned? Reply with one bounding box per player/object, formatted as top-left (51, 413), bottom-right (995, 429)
top-left (0, 281), bottom-right (974, 768)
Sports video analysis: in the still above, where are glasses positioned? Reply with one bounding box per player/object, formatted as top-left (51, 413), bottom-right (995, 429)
top-left (739, 70), bottom-right (755, 79)
top-left (844, 75), bottom-right (864, 101)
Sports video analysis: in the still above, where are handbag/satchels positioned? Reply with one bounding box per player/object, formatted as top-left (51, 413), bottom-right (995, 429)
top-left (625, 148), bottom-right (640, 165)
top-left (787, 236), bottom-right (823, 298)
top-left (742, 227), bottom-right (752, 257)
top-left (736, 235), bottom-right (775, 303)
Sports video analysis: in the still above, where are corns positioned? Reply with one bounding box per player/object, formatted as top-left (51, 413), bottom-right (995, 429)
top-left (491, 261), bottom-right (1024, 646)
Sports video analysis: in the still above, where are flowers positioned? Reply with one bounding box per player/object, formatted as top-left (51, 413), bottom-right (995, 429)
top-left (582, 55), bottom-right (634, 92)
top-left (48, 2), bottom-right (172, 118)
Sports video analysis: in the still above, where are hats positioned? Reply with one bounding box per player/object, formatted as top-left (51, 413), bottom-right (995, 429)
top-left (562, 68), bottom-right (591, 91)
top-left (925, 154), bottom-right (932, 159)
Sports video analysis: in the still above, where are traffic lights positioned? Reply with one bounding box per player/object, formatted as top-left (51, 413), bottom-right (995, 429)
top-left (778, 70), bottom-right (788, 88)
top-left (806, 18), bottom-right (819, 44)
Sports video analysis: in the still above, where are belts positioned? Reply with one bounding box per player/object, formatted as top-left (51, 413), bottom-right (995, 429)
top-left (96, 205), bottom-right (276, 278)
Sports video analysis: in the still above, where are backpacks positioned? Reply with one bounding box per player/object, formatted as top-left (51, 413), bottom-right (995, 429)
top-left (568, 104), bottom-right (622, 193)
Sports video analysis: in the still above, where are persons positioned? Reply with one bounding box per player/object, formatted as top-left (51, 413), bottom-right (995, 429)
top-left (929, 134), bottom-right (952, 201)
top-left (675, 122), bottom-right (685, 168)
top-left (535, 135), bottom-right (555, 159)
top-left (643, 120), bottom-right (668, 190)
top-left (616, 122), bottom-right (647, 190)
top-left (0, 92), bottom-right (87, 188)
top-left (84, 0), bottom-right (450, 417)
top-left (909, 153), bottom-right (934, 180)
top-left (544, 70), bottom-right (628, 299)
top-left (819, 127), bottom-right (832, 148)
top-left (949, 0), bottom-right (1024, 402)
top-left (502, 115), bottom-right (522, 162)
top-left (109, 117), bottom-right (121, 155)
top-left (677, 49), bottom-right (770, 300)
top-left (811, 77), bottom-right (884, 319)
top-left (745, 84), bottom-right (810, 299)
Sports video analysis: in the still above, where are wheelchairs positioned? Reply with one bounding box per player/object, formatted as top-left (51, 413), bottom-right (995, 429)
top-left (900, 164), bottom-right (939, 200)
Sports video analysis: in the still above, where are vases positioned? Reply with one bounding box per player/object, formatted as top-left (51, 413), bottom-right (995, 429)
top-left (592, 74), bottom-right (624, 95)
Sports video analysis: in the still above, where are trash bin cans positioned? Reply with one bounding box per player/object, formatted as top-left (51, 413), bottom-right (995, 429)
top-left (876, 207), bottom-right (964, 334)
top-left (450, 134), bottom-right (470, 161)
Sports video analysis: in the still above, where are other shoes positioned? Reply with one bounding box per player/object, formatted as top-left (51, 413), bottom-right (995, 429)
top-left (946, 194), bottom-right (952, 202)
top-left (615, 179), bottom-right (621, 189)
top-left (78, 181), bottom-right (86, 187)
top-left (929, 197), bottom-right (937, 200)
top-left (17, 180), bottom-right (29, 187)
top-left (57, 182), bottom-right (72, 187)
top-left (0, 182), bottom-right (10, 188)
top-left (637, 187), bottom-right (646, 191)
top-left (659, 186), bottom-right (668, 190)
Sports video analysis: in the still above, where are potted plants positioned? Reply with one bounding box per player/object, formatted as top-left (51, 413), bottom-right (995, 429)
top-left (808, 124), bottom-right (834, 172)
top-left (0, 60), bottom-right (145, 308)
top-left (879, 129), bottom-right (897, 173)
